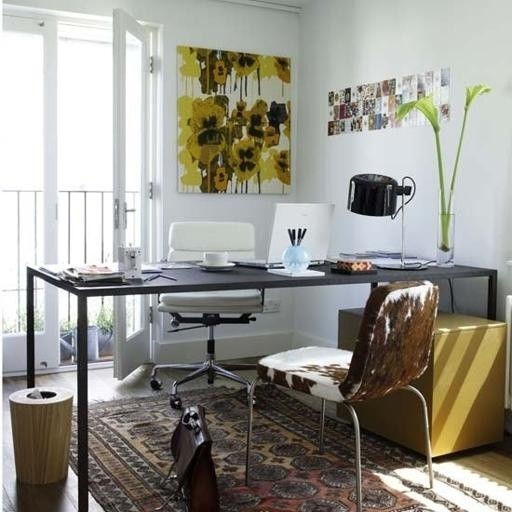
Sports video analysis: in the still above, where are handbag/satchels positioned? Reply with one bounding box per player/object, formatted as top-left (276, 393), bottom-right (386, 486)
top-left (171, 405), bottom-right (220, 511)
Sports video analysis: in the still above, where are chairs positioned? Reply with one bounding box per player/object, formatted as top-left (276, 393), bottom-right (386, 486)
top-left (152, 220), bottom-right (258, 408)
top-left (243, 276), bottom-right (440, 512)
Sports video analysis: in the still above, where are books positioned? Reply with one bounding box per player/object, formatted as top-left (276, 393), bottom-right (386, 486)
top-left (339, 250), bottom-right (417, 260)
top-left (39, 263), bottom-right (124, 285)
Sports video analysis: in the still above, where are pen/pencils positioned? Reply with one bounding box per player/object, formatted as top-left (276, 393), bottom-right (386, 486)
top-left (288, 229), bottom-right (307, 246)
top-left (143, 274), bottom-right (178, 282)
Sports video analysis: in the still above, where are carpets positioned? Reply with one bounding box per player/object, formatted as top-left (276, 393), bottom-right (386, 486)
top-left (66, 383), bottom-right (512, 512)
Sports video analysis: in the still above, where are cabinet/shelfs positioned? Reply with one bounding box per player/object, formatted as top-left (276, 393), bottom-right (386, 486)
top-left (336, 306), bottom-right (506, 460)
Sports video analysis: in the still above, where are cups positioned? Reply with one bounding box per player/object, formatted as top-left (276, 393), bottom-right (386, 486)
top-left (204, 252), bottom-right (228, 265)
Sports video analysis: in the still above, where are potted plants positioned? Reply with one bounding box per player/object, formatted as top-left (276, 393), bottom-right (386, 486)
top-left (395, 84), bottom-right (492, 268)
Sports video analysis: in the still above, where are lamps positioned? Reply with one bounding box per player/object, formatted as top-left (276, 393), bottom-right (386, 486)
top-left (346, 174), bottom-right (430, 270)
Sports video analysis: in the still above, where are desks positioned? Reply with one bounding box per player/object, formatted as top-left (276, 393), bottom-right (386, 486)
top-left (25, 259), bottom-right (497, 512)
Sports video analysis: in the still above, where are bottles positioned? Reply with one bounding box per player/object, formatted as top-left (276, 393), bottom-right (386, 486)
top-left (284, 245), bottom-right (311, 272)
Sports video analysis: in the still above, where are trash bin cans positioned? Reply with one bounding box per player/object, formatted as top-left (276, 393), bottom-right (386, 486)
top-left (9, 388), bottom-right (73, 485)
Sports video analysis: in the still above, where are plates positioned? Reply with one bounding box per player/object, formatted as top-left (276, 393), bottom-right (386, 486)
top-left (198, 261), bottom-right (237, 272)
